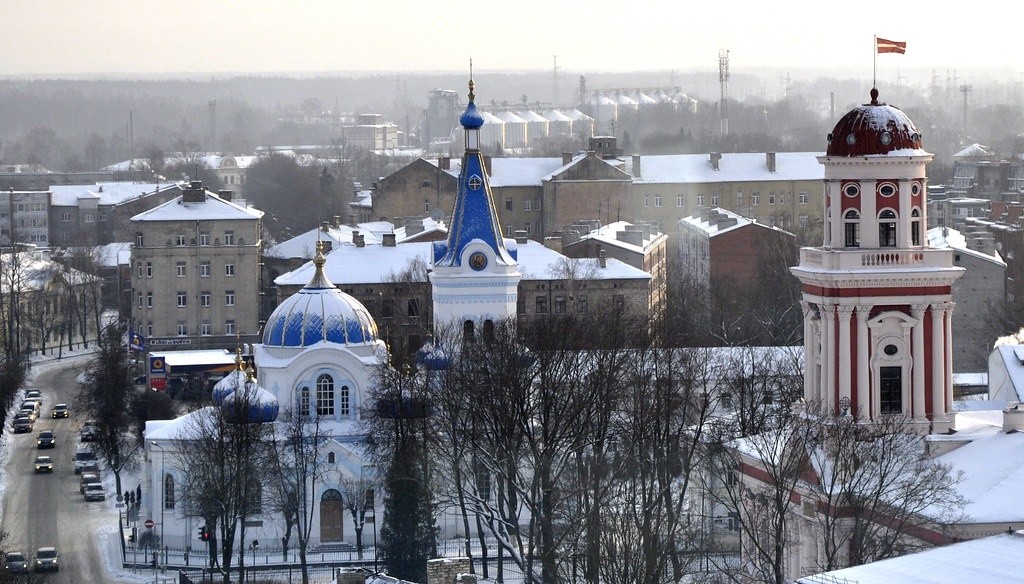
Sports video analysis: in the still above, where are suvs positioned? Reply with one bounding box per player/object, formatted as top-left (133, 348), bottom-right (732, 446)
top-left (36, 430), bottom-right (57, 450)
top-left (34, 455), bottom-right (54, 474)
top-left (32, 547), bottom-right (60, 572)
top-left (51, 403), bottom-right (70, 419)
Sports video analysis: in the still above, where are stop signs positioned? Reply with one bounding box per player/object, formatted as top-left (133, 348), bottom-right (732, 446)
top-left (144, 519), bottom-right (154, 529)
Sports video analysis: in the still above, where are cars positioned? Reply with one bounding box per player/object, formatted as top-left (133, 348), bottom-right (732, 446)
top-left (72, 421), bottom-right (106, 502)
top-left (10, 389), bottom-right (42, 434)
top-left (4, 551), bottom-right (27, 574)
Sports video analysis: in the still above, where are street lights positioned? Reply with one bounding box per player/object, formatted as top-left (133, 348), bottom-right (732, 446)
top-left (359, 464), bottom-right (378, 515)
top-left (151, 441), bottom-right (165, 573)
top-left (16, 247), bottom-right (53, 357)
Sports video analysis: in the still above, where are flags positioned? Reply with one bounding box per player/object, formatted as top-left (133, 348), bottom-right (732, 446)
top-left (877, 38), bottom-right (906, 54)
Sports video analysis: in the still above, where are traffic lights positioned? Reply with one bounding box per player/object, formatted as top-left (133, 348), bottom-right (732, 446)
top-left (149, 559), bottom-right (156, 568)
top-left (197, 525), bottom-right (205, 542)
top-left (128, 534), bottom-right (136, 543)
top-left (203, 526), bottom-right (209, 541)
